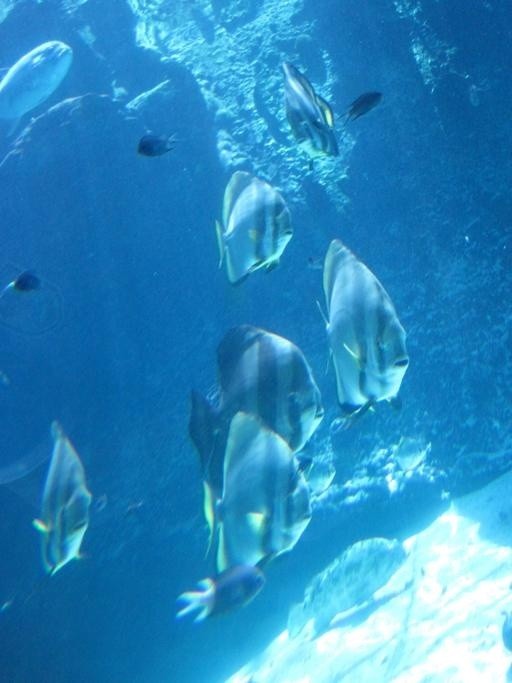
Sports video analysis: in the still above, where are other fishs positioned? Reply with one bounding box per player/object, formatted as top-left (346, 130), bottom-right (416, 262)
top-left (172, 330), bottom-right (326, 625)
